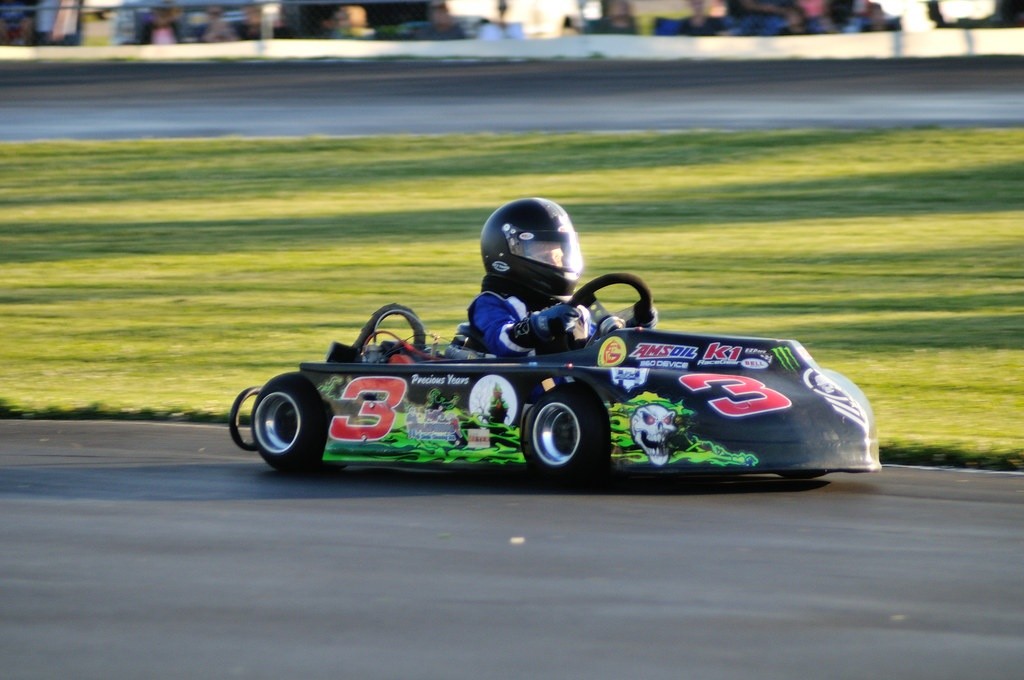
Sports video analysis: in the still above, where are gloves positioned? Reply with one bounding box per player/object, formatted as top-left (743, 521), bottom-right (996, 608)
top-left (530, 302), bottom-right (582, 341)
top-left (632, 300), bottom-right (659, 329)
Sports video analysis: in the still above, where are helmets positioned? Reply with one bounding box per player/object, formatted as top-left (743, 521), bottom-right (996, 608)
top-left (479, 197), bottom-right (583, 295)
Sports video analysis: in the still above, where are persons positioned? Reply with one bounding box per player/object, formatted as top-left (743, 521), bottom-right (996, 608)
top-left (468, 197), bottom-right (658, 358)
top-left (595, 0), bottom-right (636, 35)
top-left (198, 6), bottom-right (242, 43)
top-left (476, 0), bottom-right (525, 40)
top-left (773, 8), bottom-right (813, 35)
top-left (325, 5), bottom-right (375, 40)
top-left (249, 4), bottom-right (295, 39)
top-left (415, 2), bottom-right (466, 39)
top-left (139, 7), bottom-right (182, 44)
top-left (6, 12), bottom-right (46, 46)
top-left (858, 3), bottom-right (900, 32)
top-left (679, 0), bottom-right (732, 36)
